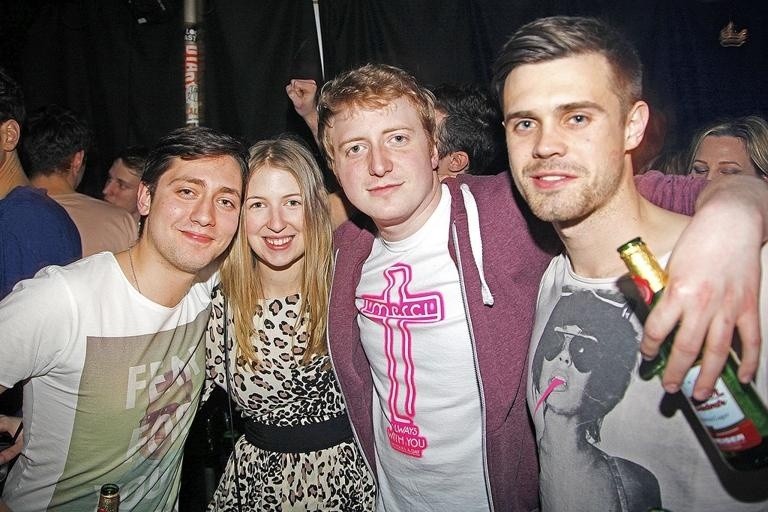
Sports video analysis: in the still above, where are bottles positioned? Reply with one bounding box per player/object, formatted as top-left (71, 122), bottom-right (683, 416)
top-left (615, 235), bottom-right (768, 479)
top-left (96, 483), bottom-right (120, 512)
